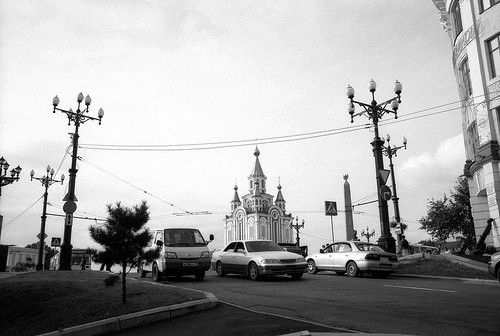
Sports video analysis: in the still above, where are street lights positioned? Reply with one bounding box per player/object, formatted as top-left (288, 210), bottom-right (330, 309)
top-left (289, 215), bottom-right (305, 247)
top-left (346, 78), bottom-right (403, 258)
top-left (360, 226), bottom-right (376, 251)
top-left (52, 92), bottom-right (104, 272)
top-left (381, 133), bottom-right (408, 242)
top-left (29, 165), bottom-right (66, 271)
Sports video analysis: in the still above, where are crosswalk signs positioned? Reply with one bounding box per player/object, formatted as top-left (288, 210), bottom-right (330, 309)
top-left (325, 201), bottom-right (338, 217)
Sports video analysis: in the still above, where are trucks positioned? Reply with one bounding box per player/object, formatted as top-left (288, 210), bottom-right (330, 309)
top-left (137, 227), bottom-right (215, 284)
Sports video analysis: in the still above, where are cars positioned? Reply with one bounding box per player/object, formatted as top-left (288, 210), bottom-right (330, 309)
top-left (211, 239), bottom-right (309, 282)
top-left (305, 240), bottom-right (401, 278)
top-left (488, 251), bottom-right (500, 282)
top-left (457, 238), bottom-right (481, 256)
